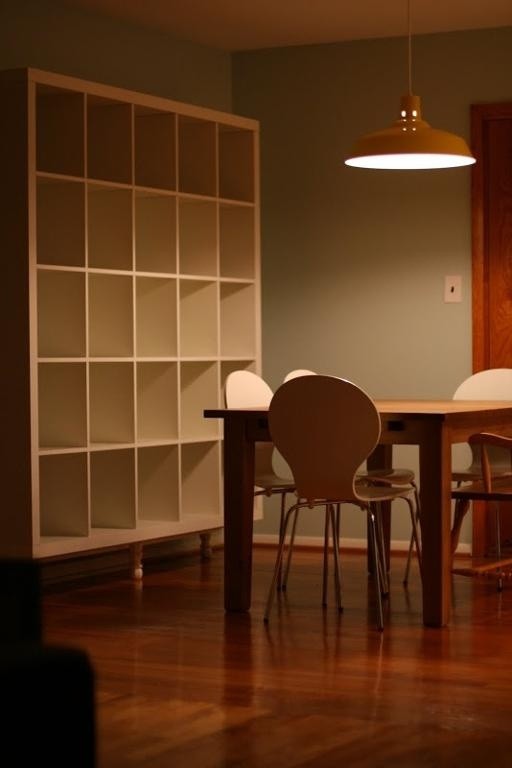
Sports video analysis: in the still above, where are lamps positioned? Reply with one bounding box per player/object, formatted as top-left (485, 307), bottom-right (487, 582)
top-left (344, 0), bottom-right (478, 170)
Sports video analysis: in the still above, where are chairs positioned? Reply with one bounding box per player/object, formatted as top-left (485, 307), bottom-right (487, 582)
top-left (402, 367), bottom-right (511, 605)
top-left (225, 367), bottom-right (429, 632)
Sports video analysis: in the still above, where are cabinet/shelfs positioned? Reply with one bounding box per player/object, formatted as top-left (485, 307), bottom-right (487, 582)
top-left (0, 67), bottom-right (263, 590)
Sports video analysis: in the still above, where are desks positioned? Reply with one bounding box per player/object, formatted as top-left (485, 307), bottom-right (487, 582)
top-left (203, 400), bottom-right (511, 628)
